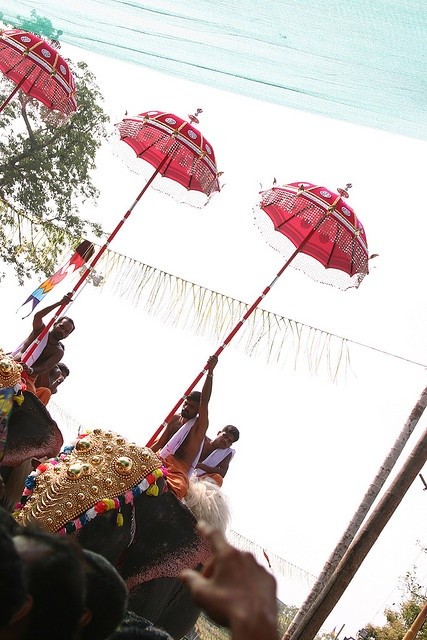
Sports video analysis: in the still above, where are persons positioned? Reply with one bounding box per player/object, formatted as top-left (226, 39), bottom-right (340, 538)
top-left (187, 425), bottom-right (239, 487)
top-left (5, 294), bottom-right (74, 394)
top-left (17, 426), bottom-right (282, 640)
top-left (35, 361), bottom-right (70, 407)
top-left (150, 355), bottom-right (218, 499)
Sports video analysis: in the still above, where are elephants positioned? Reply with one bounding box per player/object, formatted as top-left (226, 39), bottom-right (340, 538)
top-left (13, 433), bottom-right (208, 639)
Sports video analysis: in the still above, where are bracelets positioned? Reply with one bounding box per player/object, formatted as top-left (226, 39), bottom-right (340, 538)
top-left (29, 368), bottom-right (34, 375)
top-left (207, 374), bottom-right (213, 377)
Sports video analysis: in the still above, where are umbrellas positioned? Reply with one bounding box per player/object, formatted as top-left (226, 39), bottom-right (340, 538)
top-left (19, 107), bottom-right (225, 362)
top-left (0, 29), bottom-right (79, 116)
top-left (143, 177), bottom-right (379, 449)
